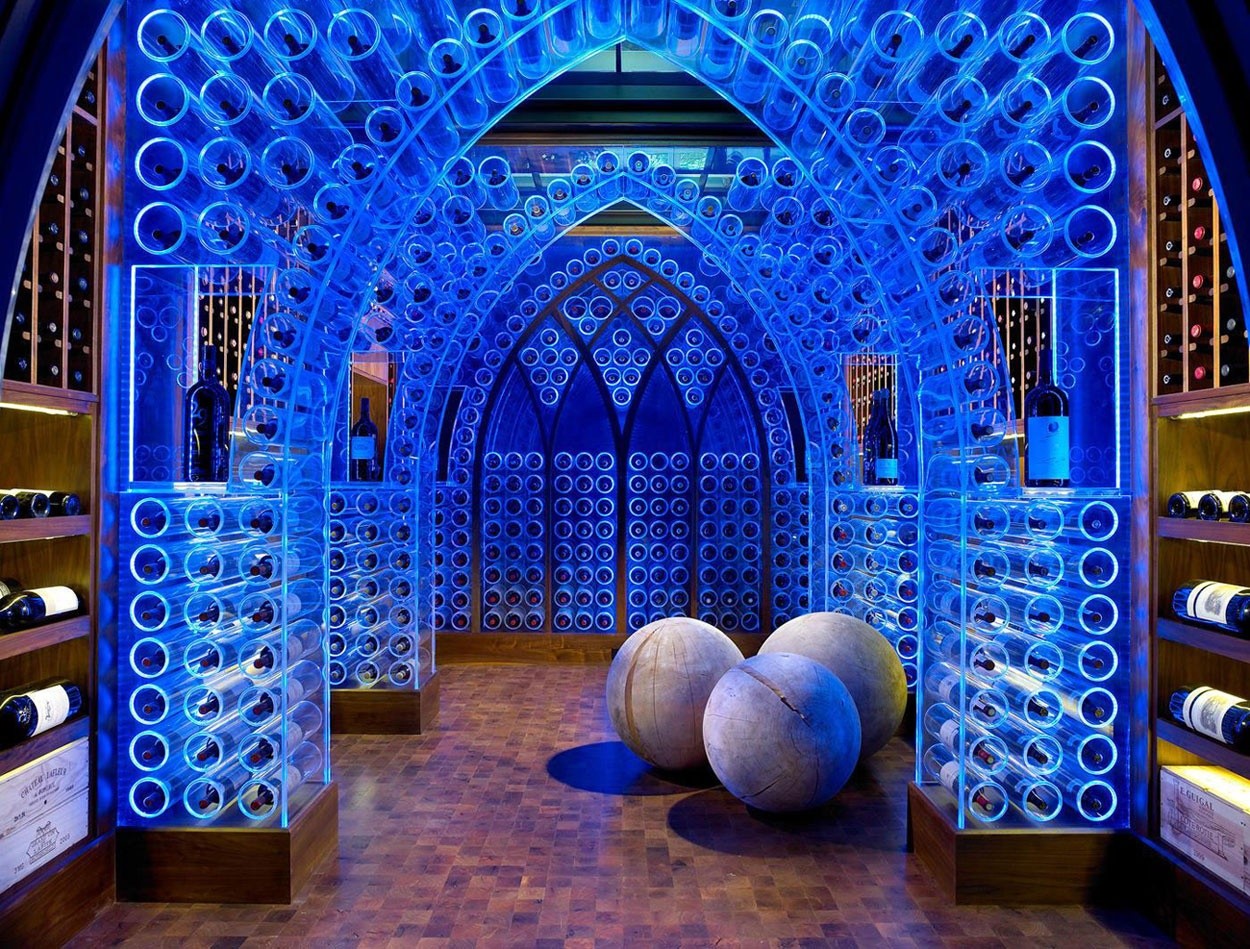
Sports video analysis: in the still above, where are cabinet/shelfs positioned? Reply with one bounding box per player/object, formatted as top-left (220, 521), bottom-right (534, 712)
top-left (1146, 383), bottom-right (1250, 949)
top-left (0, 378), bottom-right (120, 949)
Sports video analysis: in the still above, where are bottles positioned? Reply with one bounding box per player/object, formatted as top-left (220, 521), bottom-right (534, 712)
top-left (1229, 493), bottom-right (1250, 523)
top-left (1168, 683), bottom-right (1250, 755)
top-left (1198, 490), bottom-right (1247, 521)
top-left (0, 584), bottom-right (84, 636)
top-left (0, 675), bottom-right (82, 751)
top-left (0, 493), bottom-right (19, 520)
top-left (8, 91), bottom-right (95, 392)
top-left (0, 489), bottom-right (51, 518)
top-left (1173, 578), bottom-right (1250, 639)
top-left (11, 488), bottom-right (80, 516)
top-left (111, 0), bottom-right (1110, 819)
top-left (0, 577), bottom-right (23, 599)
top-left (1167, 489), bottom-right (1222, 518)
top-left (1156, 57), bottom-right (1248, 388)
